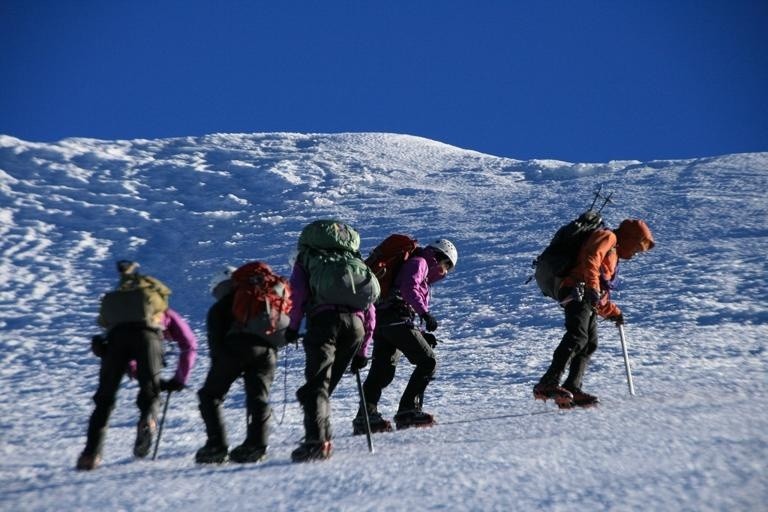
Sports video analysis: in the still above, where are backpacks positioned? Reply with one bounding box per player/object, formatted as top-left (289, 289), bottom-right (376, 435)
top-left (364, 233), bottom-right (418, 298)
top-left (534, 209), bottom-right (603, 301)
top-left (298, 220), bottom-right (381, 306)
top-left (231, 262), bottom-right (292, 350)
top-left (100, 274), bottom-right (172, 330)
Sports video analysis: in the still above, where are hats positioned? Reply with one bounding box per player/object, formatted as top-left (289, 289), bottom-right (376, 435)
top-left (641, 238), bottom-right (651, 252)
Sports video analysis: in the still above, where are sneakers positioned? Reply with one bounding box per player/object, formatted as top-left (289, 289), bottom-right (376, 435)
top-left (134, 419), bottom-right (156, 455)
top-left (289, 439), bottom-right (332, 459)
top-left (354, 405), bottom-right (391, 432)
top-left (231, 444), bottom-right (268, 463)
top-left (558, 385), bottom-right (600, 408)
top-left (534, 382), bottom-right (574, 404)
top-left (394, 409), bottom-right (432, 429)
top-left (78, 448), bottom-right (101, 469)
top-left (195, 437), bottom-right (228, 462)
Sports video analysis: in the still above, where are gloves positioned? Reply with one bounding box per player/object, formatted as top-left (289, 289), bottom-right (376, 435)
top-left (160, 377), bottom-right (183, 391)
top-left (284, 326), bottom-right (299, 344)
top-left (424, 311), bottom-right (437, 331)
top-left (609, 312), bottom-right (624, 326)
top-left (587, 288), bottom-right (601, 303)
top-left (351, 354), bottom-right (367, 374)
top-left (421, 330), bottom-right (437, 348)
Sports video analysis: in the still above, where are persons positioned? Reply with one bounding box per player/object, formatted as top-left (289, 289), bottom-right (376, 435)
top-left (531, 218), bottom-right (658, 410)
top-left (281, 251), bottom-right (378, 463)
top-left (194, 279), bottom-right (280, 466)
top-left (353, 236), bottom-right (459, 436)
top-left (74, 302), bottom-right (198, 471)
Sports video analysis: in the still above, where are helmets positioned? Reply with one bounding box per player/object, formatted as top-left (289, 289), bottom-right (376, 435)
top-left (429, 238), bottom-right (458, 267)
